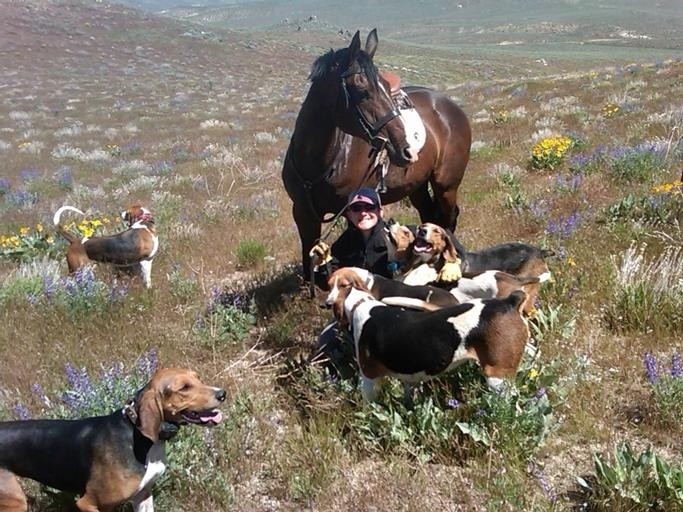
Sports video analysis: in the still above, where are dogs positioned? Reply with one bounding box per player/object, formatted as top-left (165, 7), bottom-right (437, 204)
top-left (325, 218), bottom-right (557, 411)
top-left (0, 366), bottom-right (227, 512)
top-left (53, 204), bottom-right (159, 293)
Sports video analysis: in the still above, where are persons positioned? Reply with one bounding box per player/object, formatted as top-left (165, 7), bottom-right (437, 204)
top-left (308, 186), bottom-right (402, 380)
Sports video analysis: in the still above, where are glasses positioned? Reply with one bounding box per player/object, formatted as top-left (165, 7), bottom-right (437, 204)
top-left (351, 204), bottom-right (375, 212)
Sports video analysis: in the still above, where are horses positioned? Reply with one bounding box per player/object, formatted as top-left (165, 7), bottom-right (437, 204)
top-left (280, 27), bottom-right (473, 281)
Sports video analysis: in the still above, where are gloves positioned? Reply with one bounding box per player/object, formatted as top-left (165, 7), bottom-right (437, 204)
top-left (435, 257), bottom-right (463, 283)
top-left (309, 242), bottom-right (333, 268)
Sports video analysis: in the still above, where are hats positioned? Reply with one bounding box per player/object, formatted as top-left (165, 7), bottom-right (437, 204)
top-left (346, 188), bottom-right (378, 207)
